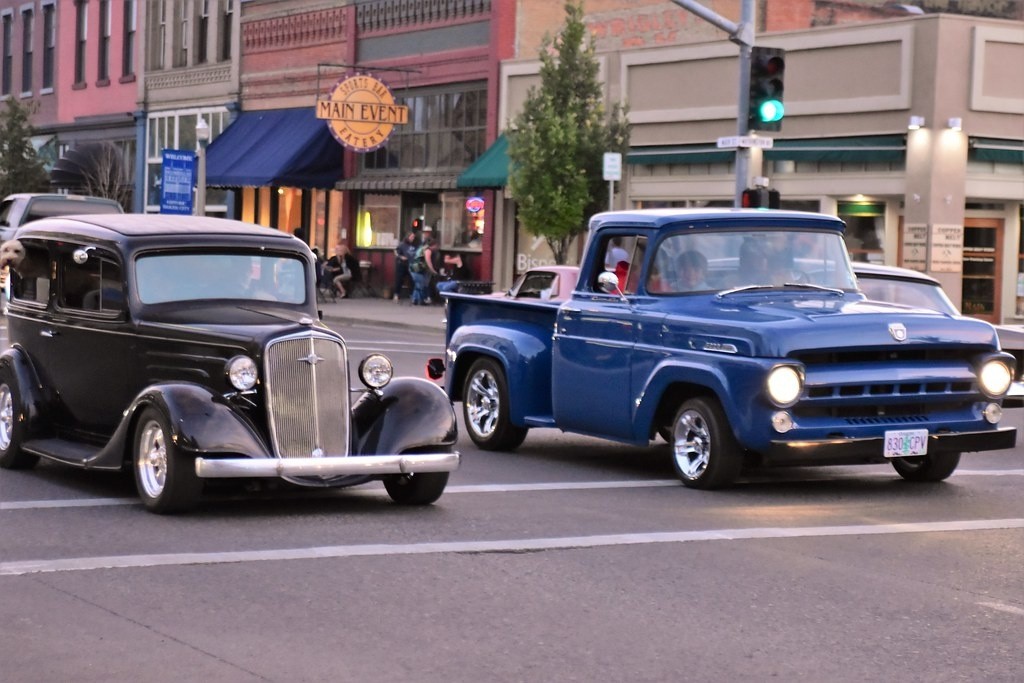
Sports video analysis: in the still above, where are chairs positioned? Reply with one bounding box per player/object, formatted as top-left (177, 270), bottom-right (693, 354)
top-left (315, 260), bottom-right (378, 304)
top-left (628, 240), bottom-right (671, 292)
top-left (81, 287), bottom-right (125, 311)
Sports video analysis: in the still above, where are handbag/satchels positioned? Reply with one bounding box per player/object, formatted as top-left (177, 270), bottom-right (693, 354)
top-left (410, 255), bottom-right (429, 272)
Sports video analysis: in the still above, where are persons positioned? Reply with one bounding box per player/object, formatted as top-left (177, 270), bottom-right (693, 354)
top-left (220, 256), bottom-right (261, 298)
top-left (293, 227), bottom-right (362, 298)
top-left (392, 232), bottom-right (474, 306)
top-left (605, 237), bottom-right (805, 295)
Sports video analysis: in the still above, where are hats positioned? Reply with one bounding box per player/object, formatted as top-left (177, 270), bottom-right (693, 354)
top-left (422, 225), bottom-right (432, 231)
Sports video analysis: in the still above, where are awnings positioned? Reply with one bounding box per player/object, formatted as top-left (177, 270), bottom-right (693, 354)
top-left (968, 137), bottom-right (1024, 165)
top-left (50, 143), bottom-right (117, 189)
top-left (457, 133), bottom-right (523, 188)
top-left (625, 135), bottom-right (906, 163)
top-left (335, 175), bottom-right (462, 193)
top-left (194, 106), bottom-right (343, 192)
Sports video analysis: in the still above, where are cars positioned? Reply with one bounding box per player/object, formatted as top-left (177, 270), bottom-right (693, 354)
top-left (0, 192), bottom-right (461, 514)
top-left (706, 259), bottom-right (1024, 407)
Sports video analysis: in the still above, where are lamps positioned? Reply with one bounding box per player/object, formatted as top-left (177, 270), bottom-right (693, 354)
top-left (948, 118), bottom-right (961, 130)
top-left (908, 115), bottom-right (926, 129)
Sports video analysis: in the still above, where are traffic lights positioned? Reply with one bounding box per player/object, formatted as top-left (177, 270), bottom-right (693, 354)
top-left (748, 46), bottom-right (786, 131)
top-left (410, 214), bottom-right (423, 232)
top-left (742, 189), bottom-right (760, 208)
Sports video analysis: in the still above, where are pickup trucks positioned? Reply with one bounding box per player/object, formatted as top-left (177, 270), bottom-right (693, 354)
top-left (426, 207), bottom-right (1017, 491)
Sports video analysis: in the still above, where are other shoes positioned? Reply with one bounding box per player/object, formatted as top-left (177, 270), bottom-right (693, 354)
top-left (341, 292), bottom-right (348, 298)
top-left (393, 294), bottom-right (400, 302)
